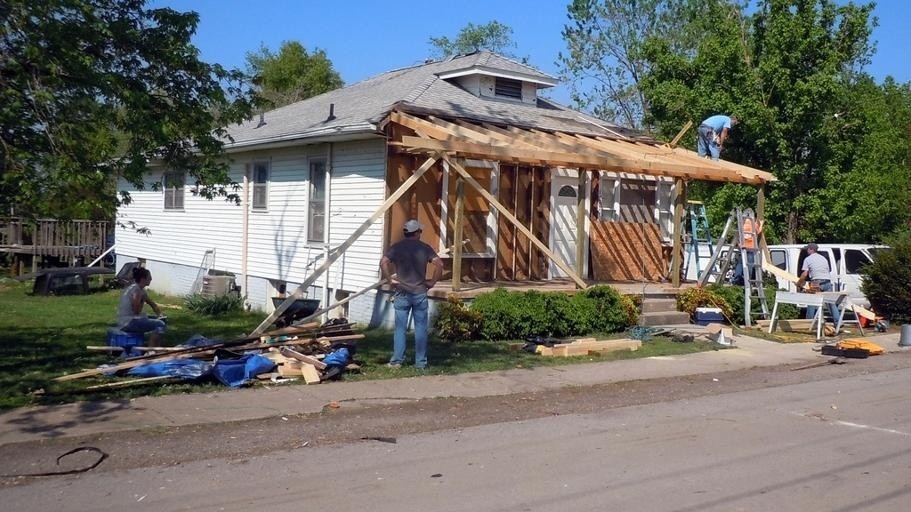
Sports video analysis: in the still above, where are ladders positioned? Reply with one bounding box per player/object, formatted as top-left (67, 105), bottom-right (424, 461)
top-left (697, 204), bottom-right (771, 328)
top-left (680, 200), bottom-right (719, 281)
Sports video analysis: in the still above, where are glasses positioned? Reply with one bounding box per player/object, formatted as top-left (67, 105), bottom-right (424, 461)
top-left (415, 230), bottom-right (422, 233)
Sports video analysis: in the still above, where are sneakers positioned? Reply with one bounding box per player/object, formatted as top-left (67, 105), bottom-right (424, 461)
top-left (386, 363), bottom-right (401, 369)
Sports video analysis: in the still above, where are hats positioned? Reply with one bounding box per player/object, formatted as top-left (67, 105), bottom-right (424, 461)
top-left (804, 243), bottom-right (818, 249)
top-left (403, 219), bottom-right (425, 232)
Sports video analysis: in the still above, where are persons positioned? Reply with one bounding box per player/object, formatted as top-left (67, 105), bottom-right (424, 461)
top-left (119, 268), bottom-right (169, 354)
top-left (732, 218), bottom-right (764, 285)
top-left (380, 221), bottom-right (444, 369)
top-left (697, 114), bottom-right (737, 161)
top-left (794, 243), bottom-right (844, 328)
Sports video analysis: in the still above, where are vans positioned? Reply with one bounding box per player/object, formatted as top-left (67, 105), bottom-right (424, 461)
top-left (761, 244), bottom-right (896, 319)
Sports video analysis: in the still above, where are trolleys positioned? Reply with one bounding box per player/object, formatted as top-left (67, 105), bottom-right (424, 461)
top-left (271, 296), bottom-right (320, 328)
